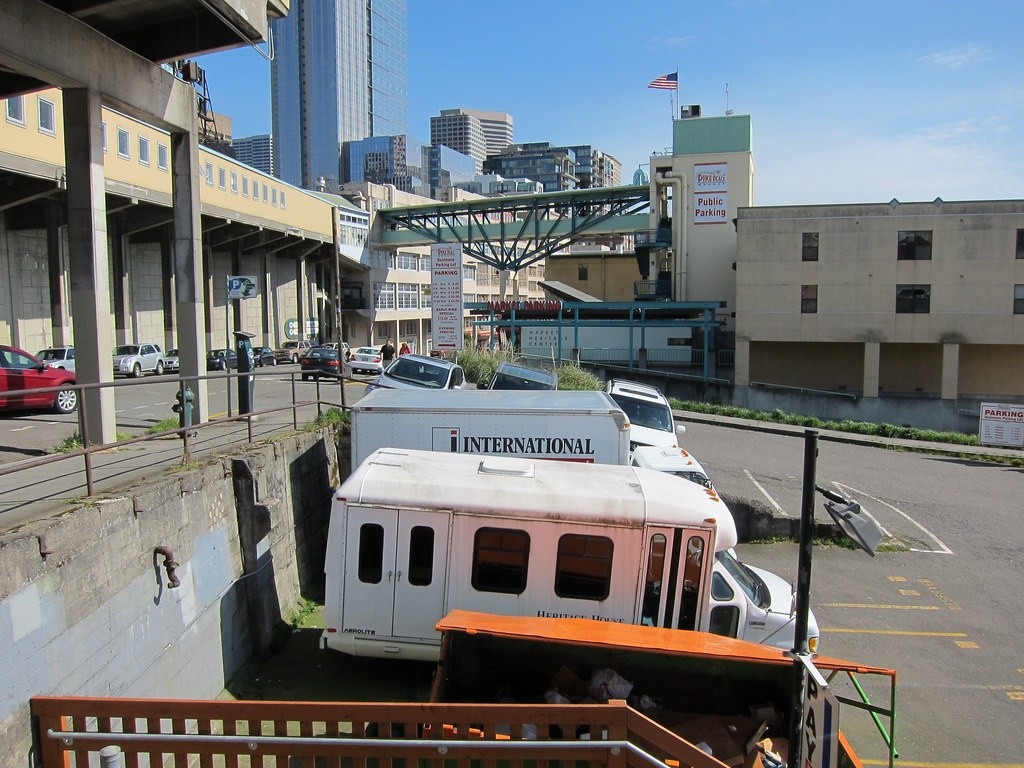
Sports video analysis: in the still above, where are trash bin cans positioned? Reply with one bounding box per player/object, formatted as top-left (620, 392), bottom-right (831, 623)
top-left (431, 350), bottom-right (441, 359)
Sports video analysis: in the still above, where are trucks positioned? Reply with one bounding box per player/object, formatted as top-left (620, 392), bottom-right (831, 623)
top-left (318, 443), bottom-right (819, 664)
top-left (350, 387), bottom-right (720, 499)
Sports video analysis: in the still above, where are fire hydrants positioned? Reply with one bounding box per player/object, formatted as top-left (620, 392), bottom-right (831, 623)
top-left (173, 386), bottom-right (195, 438)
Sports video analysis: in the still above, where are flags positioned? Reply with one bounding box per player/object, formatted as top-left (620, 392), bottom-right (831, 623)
top-left (649, 71), bottom-right (678, 91)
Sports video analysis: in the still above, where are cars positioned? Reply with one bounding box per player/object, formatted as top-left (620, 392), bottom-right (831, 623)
top-left (253, 346), bottom-right (277, 367)
top-left (0, 344), bottom-right (79, 413)
top-left (165, 349), bottom-right (179, 372)
top-left (362, 352), bottom-right (469, 395)
top-left (301, 347), bottom-right (353, 382)
top-left (476, 360), bottom-right (559, 392)
top-left (29, 345), bottom-right (76, 373)
top-left (352, 346), bottom-right (397, 373)
top-left (207, 349), bottom-right (237, 371)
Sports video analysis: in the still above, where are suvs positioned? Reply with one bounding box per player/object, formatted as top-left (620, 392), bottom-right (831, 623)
top-left (110, 344), bottom-right (166, 378)
top-left (604, 377), bottom-right (686, 451)
top-left (273, 342), bottom-right (311, 363)
top-left (321, 342), bottom-right (350, 356)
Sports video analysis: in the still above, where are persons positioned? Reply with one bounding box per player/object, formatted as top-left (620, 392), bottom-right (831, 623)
top-left (399, 343), bottom-right (410, 357)
top-left (380, 339), bottom-right (395, 370)
